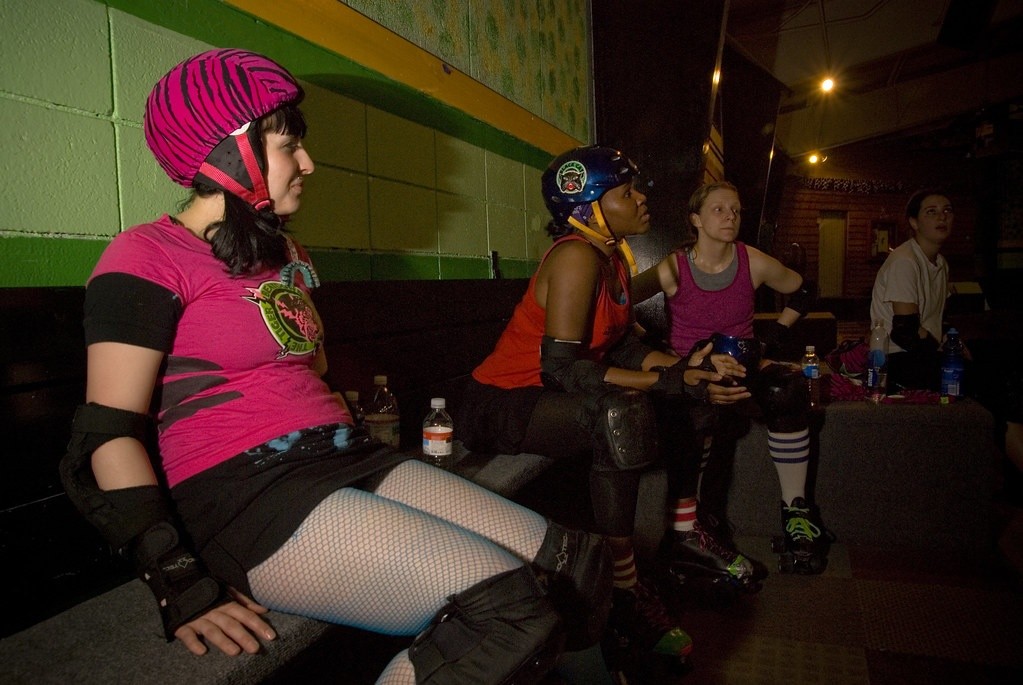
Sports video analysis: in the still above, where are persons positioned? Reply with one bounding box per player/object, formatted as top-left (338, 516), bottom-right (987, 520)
top-left (80, 48), bottom-right (615, 684)
top-left (453, 145), bottom-right (752, 660)
top-left (631, 181), bottom-right (822, 572)
top-left (869, 186), bottom-right (1023, 473)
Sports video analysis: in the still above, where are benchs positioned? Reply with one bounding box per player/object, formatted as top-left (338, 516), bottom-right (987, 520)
top-left (635, 397), bottom-right (990, 547)
top-left (0, 277), bottom-right (546, 685)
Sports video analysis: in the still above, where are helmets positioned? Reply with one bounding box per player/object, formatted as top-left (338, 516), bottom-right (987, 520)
top-left (825, 340), bottom-right (870, 374)
top-left (690, 334), bottom-right (760, 367)
top-left (145, 49), bottom-right (304, 189)
top-left (540, 145), bottom-right (642, 226)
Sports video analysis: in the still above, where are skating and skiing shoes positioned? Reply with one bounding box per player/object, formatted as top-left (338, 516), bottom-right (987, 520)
top-left (770, 496), bottom-right (823, 575)
top-left (658, 526), bottom-right (755, 605)
top-left (609, 585), bottom-right (693, 677)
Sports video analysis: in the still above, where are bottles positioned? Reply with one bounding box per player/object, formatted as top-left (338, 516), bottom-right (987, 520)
top-left (345, 391), bottom-right (364, 430)
top-left (866, 318), bottom-right (890, 407)
top-left (365, 376), bottom-right (400, 450)
top-left (801, 346), bottom-right (820, 407)
top-left (939, 327), bottom-right (970, 405)
top-left (422, 398), bottom-right (454, 470)
